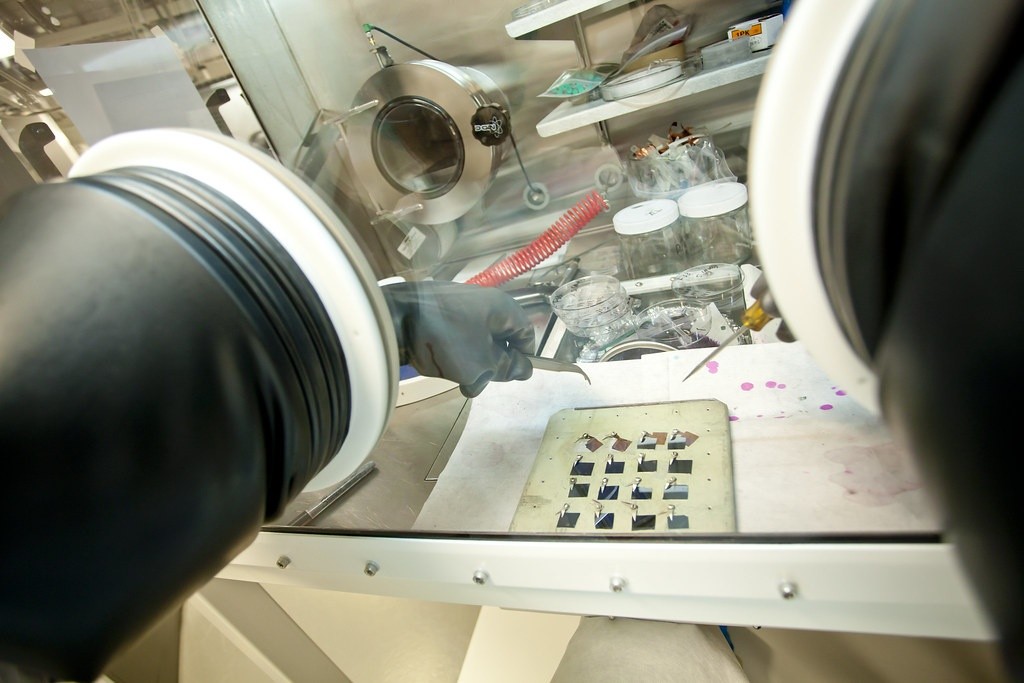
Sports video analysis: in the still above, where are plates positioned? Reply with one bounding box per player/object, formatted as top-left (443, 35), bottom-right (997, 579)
top-left (511, 0), bottom-right (568, 22)
top-left (633, 297), bottom-right (713, 348)
top-left (550, 274), bottom-right (632, 337)
top-left (699, 35), bottom-right (751, 70)
top-left (599, 53), bottom-right (702, 102)
top-left (670, 262), bottom-right (745, 302)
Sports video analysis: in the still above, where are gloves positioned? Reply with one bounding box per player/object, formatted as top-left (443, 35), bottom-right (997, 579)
top-left (380, 281), bottom-right (536, 398)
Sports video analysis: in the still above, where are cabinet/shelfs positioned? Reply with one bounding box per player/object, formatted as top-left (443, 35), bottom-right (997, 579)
top-left (504, 1), bottom-right (771, 140)
